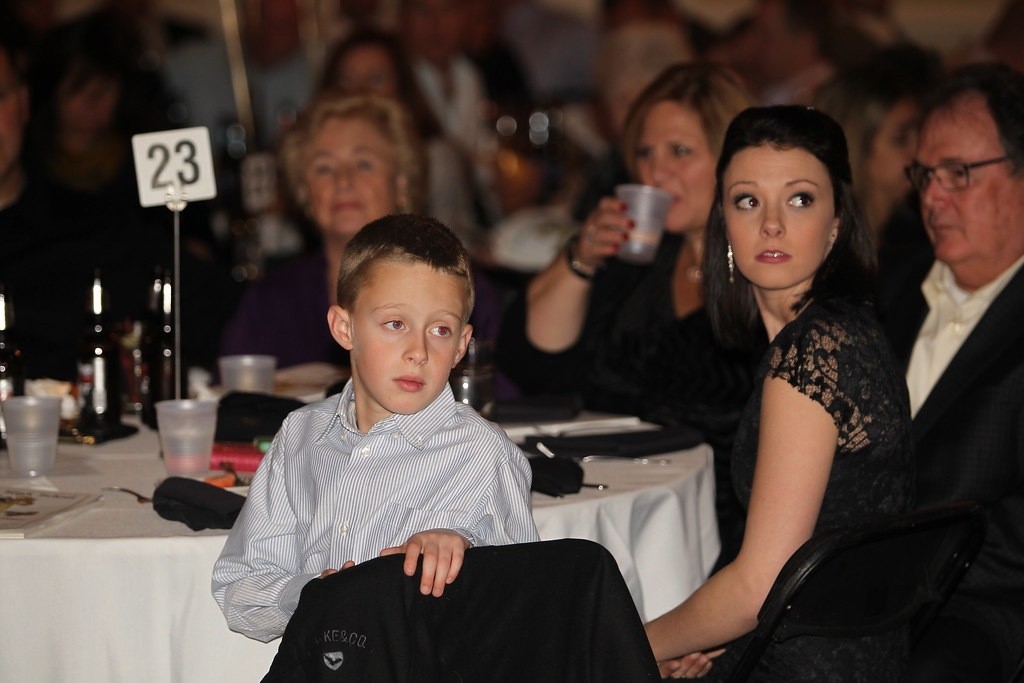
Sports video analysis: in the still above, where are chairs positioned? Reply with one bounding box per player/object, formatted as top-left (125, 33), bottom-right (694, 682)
top-left (260, 538), bottom-right (661, 683)
top-left (706, 499), bottom-right (990, 683)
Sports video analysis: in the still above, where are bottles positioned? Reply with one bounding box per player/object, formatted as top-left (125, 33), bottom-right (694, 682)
top-left (142, 263), bottom-right (177, 430)
top-left (0, 291), bottom-right (25, 449)
top-left (75, 270), bottom-right (122, 438)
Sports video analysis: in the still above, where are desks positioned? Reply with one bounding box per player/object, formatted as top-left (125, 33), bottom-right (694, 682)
top-left (0, 357), bottom-right (722, 683)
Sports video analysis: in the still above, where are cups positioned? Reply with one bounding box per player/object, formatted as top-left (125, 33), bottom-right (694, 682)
top-left (611, 184), bottom-right (674, 262)
top-left (153, 398), bottom-right (222, 481)
top-left (214, 354), bottom-right (280, 405)
top-left (0, 393), bottom-right (62, 479)
top-left (448, 338), bottom-right (497, 420)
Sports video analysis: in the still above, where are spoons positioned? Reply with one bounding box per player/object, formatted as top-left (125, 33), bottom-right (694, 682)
top-left (97, 487), bottom-right (156, 505)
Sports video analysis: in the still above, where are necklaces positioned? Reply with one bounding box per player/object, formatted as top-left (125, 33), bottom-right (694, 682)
top-left (687, 266), bottom-right (702, 283)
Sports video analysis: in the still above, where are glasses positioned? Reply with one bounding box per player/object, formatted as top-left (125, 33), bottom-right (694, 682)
top-left (904, 150), bottom-right (1024, 193)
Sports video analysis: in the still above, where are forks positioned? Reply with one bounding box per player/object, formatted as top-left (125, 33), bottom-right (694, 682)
top-left (534, 441), bottom-right (673, 469)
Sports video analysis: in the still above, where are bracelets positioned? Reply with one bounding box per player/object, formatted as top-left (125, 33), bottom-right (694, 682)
top-left (563, 236), bottom-right (596, 282)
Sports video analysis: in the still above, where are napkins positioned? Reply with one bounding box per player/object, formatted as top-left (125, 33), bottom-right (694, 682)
top-left (152, 476), bottom-right (246, 532)
top-left (215, 389), bottom-right (306, 441)
top-left (528, 457), bottom-right (586, 497)
top-left (519, 422), bottom-right (707, 460)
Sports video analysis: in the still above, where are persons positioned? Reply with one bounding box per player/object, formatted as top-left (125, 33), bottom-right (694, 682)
top-left (209, 210), bottom-right (545, 642)
top-left (0, 0), bottom-right (1024, 681)
